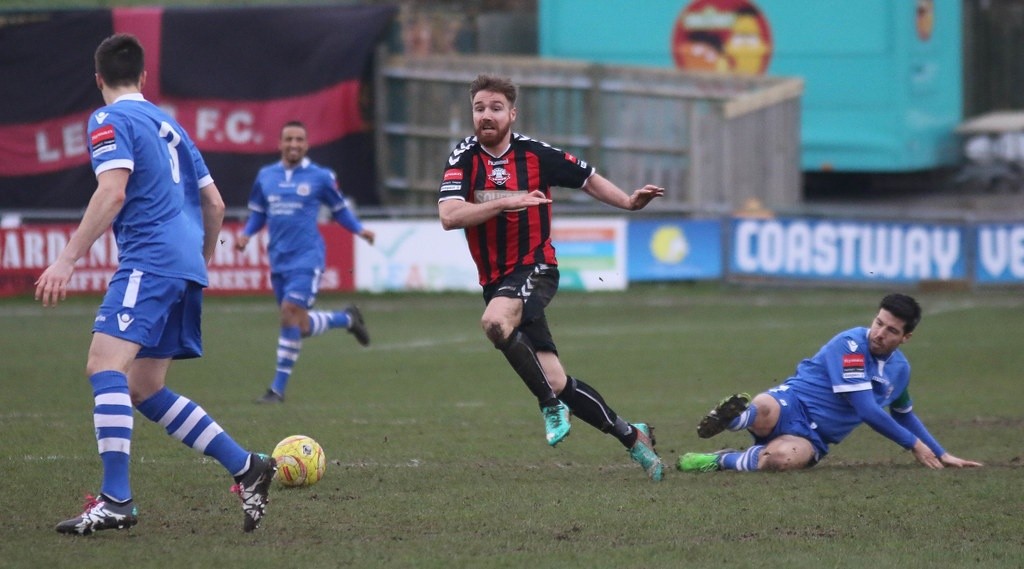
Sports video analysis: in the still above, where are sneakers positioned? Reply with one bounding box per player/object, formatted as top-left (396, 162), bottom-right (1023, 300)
top-left (55, 492), bottom-right (139, 535)
top-left (230, 453), bottom-right (277, 533)
top-left (541, 399), bottom-right (571, 448)
top-left (345, 306), bottom-right (369, 347)
top-left (676, 448), bottom-right (736, 474)
top-left (626, 423), bottom-right (664, 483)
top-left (253, 387), bottom-right (283, 405)
top-left (697, 392), bottom-right (752, 439)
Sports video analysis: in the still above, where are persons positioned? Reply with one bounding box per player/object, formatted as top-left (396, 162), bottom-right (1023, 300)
top-left (33, 32), bottom-right (276, 537)
top-left (675, 293), bottom-right (984, 472)
top-left (437, 74), bottom-right (667, 483)
top-left (236, 121), bottom-right (375, 404)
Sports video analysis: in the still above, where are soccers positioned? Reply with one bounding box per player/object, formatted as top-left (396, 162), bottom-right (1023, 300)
top-left (271, 435), bottom-right (326, 487)
